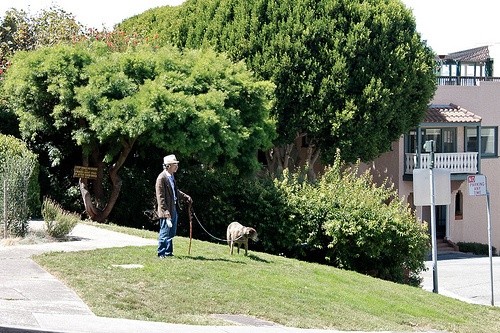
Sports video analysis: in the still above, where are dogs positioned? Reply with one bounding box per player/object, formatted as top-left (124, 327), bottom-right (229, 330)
top-left (226, 221), bottom-right (259, 256)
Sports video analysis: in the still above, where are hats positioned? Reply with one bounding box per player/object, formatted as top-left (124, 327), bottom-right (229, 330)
top-left (163, 154), bottom-right (179, 164)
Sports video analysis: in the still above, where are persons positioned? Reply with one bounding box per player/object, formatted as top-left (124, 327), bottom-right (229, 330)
top-left (155, 155), bottom-right (193, 258)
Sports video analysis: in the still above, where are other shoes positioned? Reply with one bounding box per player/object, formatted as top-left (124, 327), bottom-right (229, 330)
top-left (159, 254), bottom-right (166, 259)
